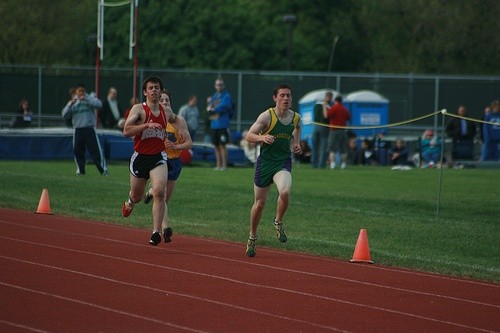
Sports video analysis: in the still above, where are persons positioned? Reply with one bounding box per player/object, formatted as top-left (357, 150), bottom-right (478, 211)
top-left (61, 84), bottom-right (110, 177)
top-left (245, 83), bottom-right (304, 258)
top-left (420, 127), bottom-right (448, 168)
top-left (322, 95), bottom-right (352, 170)
top-left (477, 100), bottom-right (500, 162)
top-left (142, 88), bottom-right (193, 244)
top-left (204, 77), bottom-right (233, 171)
top-left (310, 91), bottom-right (334, 170)
top-left (122, 75), bottom-right (187, 245)
top-left (288, 135), bottom-right (312, 165)
top-left (178, 93), bottom-right (205, 168)
top-left (347, 131), bottom-right (410, 167)
top-left (98, 88), bottom-right (125, 129)
top-left (445, 106), bottom-right (477, 169)
top-left (8, 99), bottom-right (33, 129)
top-left (124, 95), bottom-right (140, 120)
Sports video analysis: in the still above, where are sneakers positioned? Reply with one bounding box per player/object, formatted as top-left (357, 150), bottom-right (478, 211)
top-left (123, 199), bottom-right (135, 217)
top-left (164, 227), bottom-right (172, 243)
top-left (149, 232), bottom-right (161, 246)
top-left (144, 191), bottom-right (152, 204)
top-left (246, 237), bottom-right (257, 256)
top-left (272, 217), bottom-right (287, 243)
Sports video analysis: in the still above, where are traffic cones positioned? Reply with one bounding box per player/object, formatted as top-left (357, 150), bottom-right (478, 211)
top-left (349, 228), bottom-right (374, 264)
top-left (34, 188), bottom-right (54, 215)
